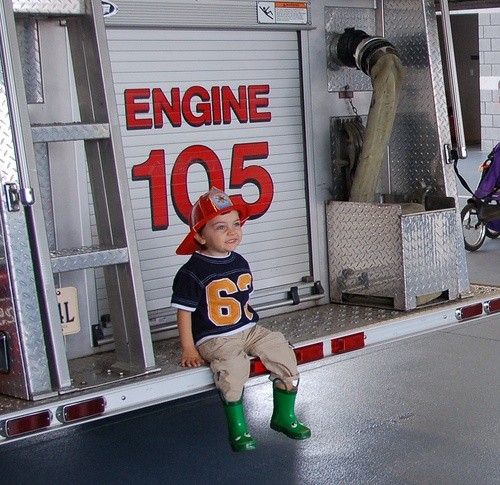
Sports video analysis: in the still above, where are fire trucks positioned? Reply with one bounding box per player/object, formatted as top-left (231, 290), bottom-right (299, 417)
top-left (0, 0), bottom-right (499, 454)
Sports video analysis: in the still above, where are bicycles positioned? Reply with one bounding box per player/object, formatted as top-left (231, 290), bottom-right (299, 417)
top-left (461, 140), bottom-right (500, 251)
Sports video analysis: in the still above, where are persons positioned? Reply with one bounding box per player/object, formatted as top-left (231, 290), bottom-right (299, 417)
top-left (168, 187), bottom-right (312, 454)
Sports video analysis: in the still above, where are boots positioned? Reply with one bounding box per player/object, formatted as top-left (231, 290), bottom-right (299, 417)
top-left (217, 388), bottom-right (257, 451)
top-left (269, 376), bottom-right (311, 441)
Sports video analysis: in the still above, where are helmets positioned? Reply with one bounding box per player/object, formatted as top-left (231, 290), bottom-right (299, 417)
top-left (175, 187), bottom-right (249, 255)
